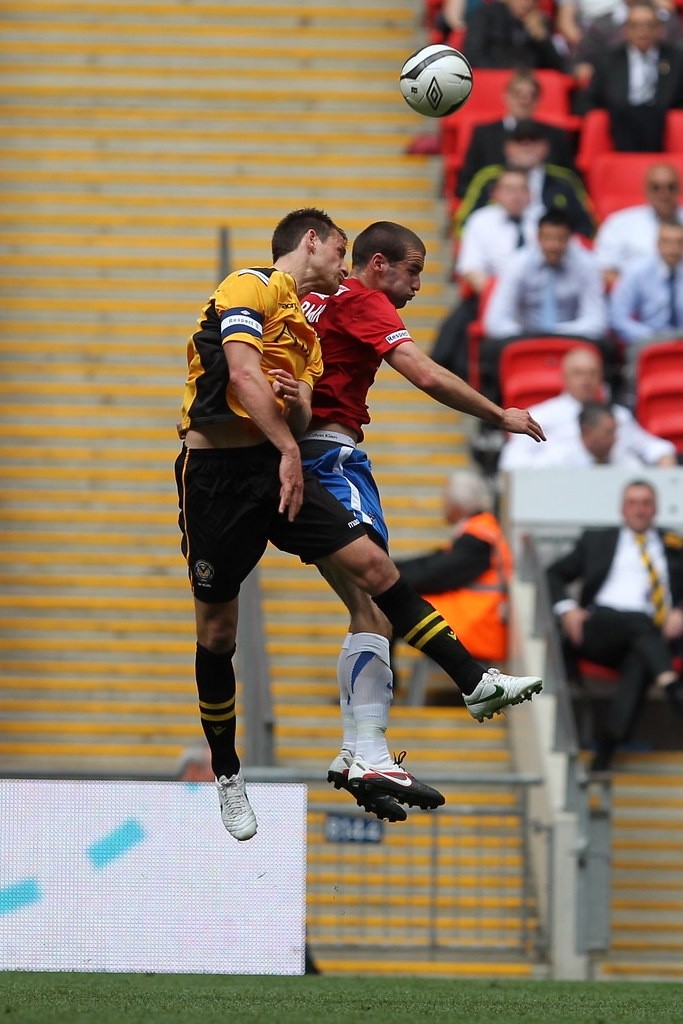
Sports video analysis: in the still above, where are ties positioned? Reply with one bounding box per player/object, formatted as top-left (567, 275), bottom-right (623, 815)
top-left (669, 267), bottom-right (678, 327)
top-left (637, 533), bottom-right (667, 625)
top-left (512, 215), bottom-right (525, 248)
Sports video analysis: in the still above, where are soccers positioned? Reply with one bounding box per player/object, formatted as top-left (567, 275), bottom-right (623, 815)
top-left (398, 43), bottom-right (476, 119)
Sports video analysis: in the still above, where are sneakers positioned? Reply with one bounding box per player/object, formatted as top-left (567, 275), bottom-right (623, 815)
top-left (347, 751), bottom-right (447, 809)
top-left (214, 765), bottom-right (257, 842)
top-left (326, 746), bottom-right (407, 822)
top-left (463, 669), bottom-right (543, 724)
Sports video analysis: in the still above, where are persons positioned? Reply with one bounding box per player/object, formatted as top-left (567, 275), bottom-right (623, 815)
top-left (435, 0), bottom-right (683, 110)
top-left (296, 220), bottom-right (548, 825)
top-left (327, 472), bottom-right (512, 708)
top-left (172, 209), bottom-right (546, 843)
top-left (543, 479), bottom-right (683, 774)
top-left (498, 345), bottom-right (679, 528)
top-left (429, 72), bottom-right (683, 443)
top-left (566, 7), bottom-right (683, 152)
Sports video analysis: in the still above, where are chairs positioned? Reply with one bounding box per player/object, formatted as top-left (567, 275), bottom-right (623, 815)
top-left (426, 0), bottom-right (683, 747)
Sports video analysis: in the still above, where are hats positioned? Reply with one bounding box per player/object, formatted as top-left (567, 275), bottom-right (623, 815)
top-left (499, 119), bottom-right (547, 143)
